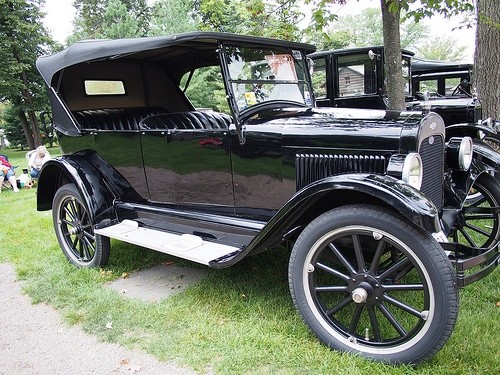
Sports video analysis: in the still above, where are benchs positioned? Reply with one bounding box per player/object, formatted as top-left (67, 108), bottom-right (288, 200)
top-left (139, 110), bottom-right (234, 130)
top-left (72, 103), bottom-right (171, 131)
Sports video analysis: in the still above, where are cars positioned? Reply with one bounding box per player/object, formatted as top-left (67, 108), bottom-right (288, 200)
top-left (36, 31), bottom-right (500, 365)
top-left (306, 46), bottom-right (500, 204)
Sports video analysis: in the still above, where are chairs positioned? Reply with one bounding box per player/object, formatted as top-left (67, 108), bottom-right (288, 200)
top-left (26, 150), bottom-right (50, 189)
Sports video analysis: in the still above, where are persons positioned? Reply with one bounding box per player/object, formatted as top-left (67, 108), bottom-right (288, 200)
top-left (28, 145), bottom-right (51, 183)
top-left (0, 156), bottom-right (19, 194)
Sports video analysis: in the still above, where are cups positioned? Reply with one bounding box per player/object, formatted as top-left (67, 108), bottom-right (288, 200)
top-left (16, 180), bottom-right (20, 189)
top-left (20, 182), bottom-right (24, 188)
